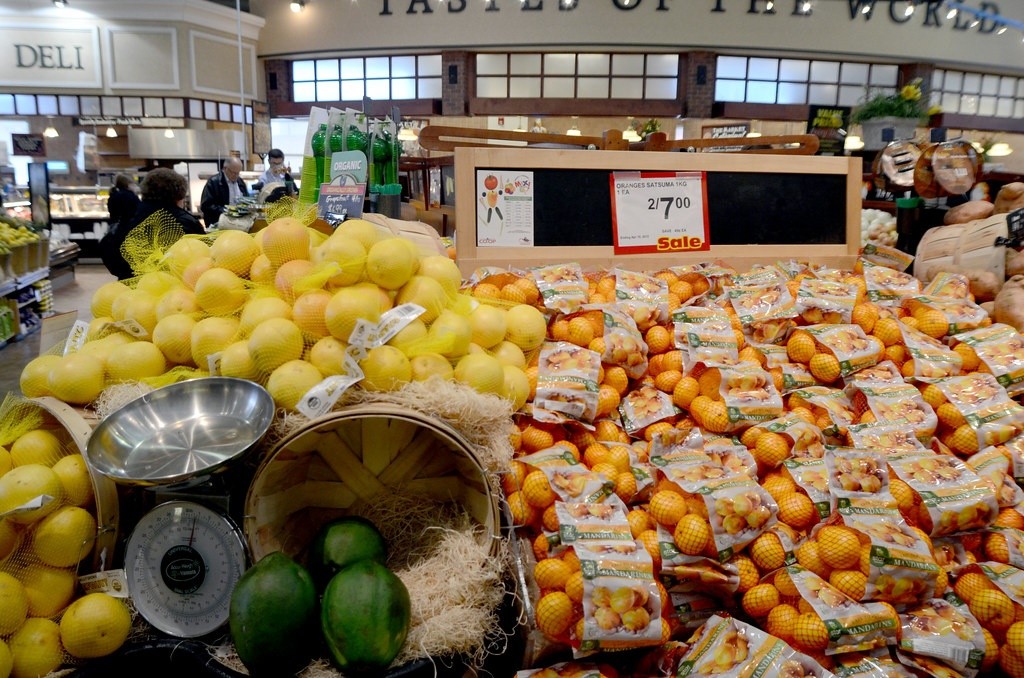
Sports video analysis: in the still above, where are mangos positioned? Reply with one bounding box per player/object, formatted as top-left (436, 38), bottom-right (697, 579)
top-left (0, 222), bottom-right (41, 256)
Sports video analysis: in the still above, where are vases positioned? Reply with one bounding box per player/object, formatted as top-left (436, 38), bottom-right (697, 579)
top-left (929, 113), bottom-right (944, 128)
top-left (859, 116), bottom-right (919, 150)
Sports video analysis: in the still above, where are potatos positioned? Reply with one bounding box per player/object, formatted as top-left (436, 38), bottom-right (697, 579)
top-left (866, 222), bottom-right (900, 250)
top-left (925, 182), bottom-right (1024, 341)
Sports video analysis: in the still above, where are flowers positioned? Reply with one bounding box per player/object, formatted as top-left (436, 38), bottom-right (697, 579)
top-left (848, 84), bottom-right (941, 130)
top-left (637, 117), bottom-right (663, 138)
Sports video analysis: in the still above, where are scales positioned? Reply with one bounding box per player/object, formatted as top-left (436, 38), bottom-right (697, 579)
top-left (83, 376), bottom-right (276, 641)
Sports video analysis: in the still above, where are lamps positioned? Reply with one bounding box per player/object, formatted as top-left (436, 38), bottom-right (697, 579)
top-left (43, 118), bottom-right (59, 137)
top-left (164, 120), bottom-right (174, 138)
top-left (289, 0), bottom-right (304, 13)
top-left (105, 120), bottom-right (118, 137)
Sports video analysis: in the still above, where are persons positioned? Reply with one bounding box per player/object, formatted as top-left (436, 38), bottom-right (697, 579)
top-left (107, 174), bottom-right (141, 223)
top-left (259, 148), bottom-right (299, 196)
top-left (201, 157), bottom-right (249, 228)
top-left (99, 167), bottom-right (206, 281)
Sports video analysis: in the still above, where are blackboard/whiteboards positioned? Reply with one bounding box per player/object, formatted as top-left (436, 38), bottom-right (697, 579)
top-left (319, 173), bottom-right (367, 219)
top-left (701, 122), bottom-right (749, 153)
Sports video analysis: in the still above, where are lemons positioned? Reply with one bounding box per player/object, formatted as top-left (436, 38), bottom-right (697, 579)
top-left (0, 430), bottom-right (132, 678)
top-left (20, 217), bottom-right (548, 419)
top-left (475, 269), bottom-right (1024, 678)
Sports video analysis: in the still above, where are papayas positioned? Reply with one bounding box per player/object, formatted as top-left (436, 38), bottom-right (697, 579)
top-left (228, 514), bottom-right (411, 678)
